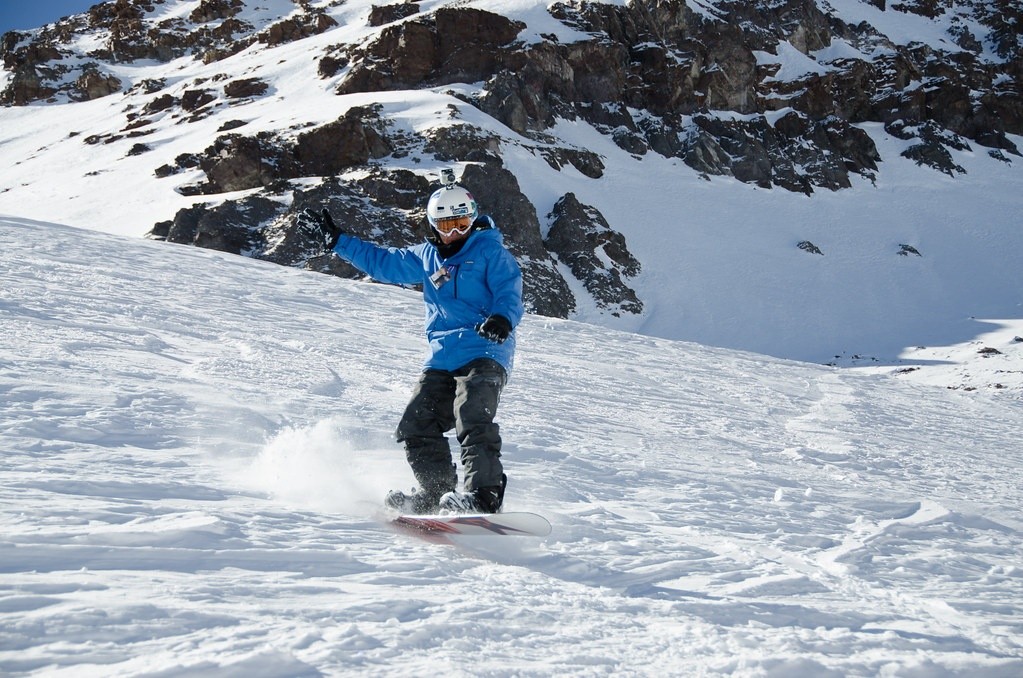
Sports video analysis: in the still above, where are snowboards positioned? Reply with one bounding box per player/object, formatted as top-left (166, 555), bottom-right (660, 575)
top-left (392, 512), bottom-right (553, 539)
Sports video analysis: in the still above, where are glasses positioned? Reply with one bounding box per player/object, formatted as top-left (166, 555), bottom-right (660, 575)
top-left (430, 214), bottom-right (472, 234)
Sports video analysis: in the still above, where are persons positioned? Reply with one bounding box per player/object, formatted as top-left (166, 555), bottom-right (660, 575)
top-left (297, 185), bottom-right (524, 513)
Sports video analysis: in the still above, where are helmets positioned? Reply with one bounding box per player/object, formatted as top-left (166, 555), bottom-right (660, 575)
top-left (426, 184), bottom-right (478, 240)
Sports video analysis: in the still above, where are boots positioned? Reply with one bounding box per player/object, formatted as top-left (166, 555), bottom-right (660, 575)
top-left (385, 487), bottom-right (449, 513)
top-left (439, 471), bottom-right (507, 514)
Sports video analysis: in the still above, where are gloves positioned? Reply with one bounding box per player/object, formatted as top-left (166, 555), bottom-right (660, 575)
top-left (477, 314), bottom-right (512, 343)
top-left (297, 206), bottom-right (342, 250)
